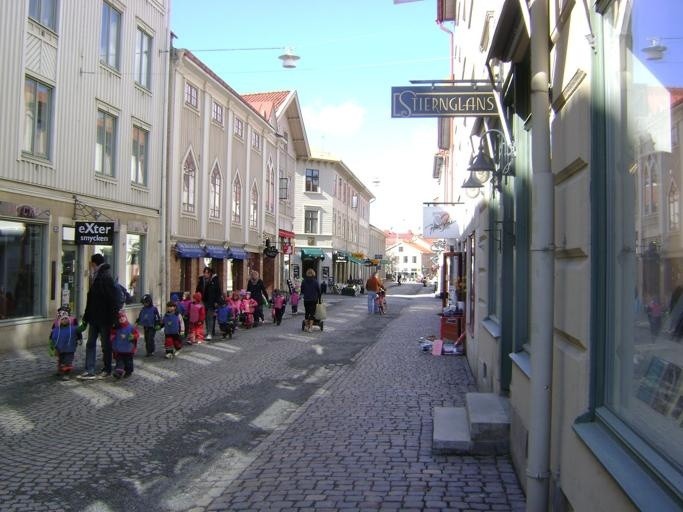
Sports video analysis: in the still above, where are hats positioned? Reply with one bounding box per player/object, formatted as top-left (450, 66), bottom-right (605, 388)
top-left (117, 311), bottom-right (127, 319)
top-left (56, 303), bottom-right (71, 326)
top-left (193, 293), bottom-right (201, 302)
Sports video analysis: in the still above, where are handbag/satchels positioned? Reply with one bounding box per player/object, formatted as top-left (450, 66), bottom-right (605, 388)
top-left (261, 294), bottom-right (268, 306)
top-left (315, 303), bottom-right (327, 319)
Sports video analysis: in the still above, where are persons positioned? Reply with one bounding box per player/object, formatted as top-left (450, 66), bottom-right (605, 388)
top-left (365, 272), bottom-right (386, 315)
top-left (620, 237), bottom-right (663, 343)
top-left (397, 272), bottom-right (401, 286)
top-left (289, 291), bottom-right (301, 315)
top-left (3, 264), bottom-right (33, 320)
top-left (299, 269), bottom-right (322, 333)
top-left (49, 254), bottom-right (286, 379)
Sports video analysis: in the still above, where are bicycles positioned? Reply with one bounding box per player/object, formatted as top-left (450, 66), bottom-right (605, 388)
top-left (376, 287), bottom-right (388, 316)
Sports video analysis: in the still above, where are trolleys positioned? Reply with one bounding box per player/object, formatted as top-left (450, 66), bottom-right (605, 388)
top-left (302, 291), bottom-right (325, 331)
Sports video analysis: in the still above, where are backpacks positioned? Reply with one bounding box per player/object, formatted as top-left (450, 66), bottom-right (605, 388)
top-left (115, 281), bottom-right (126, 309)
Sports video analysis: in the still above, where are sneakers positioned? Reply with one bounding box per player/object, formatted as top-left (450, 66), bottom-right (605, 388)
top-left (98, 368), bottom-right (112, 379)
top-left (124, 372), bottom-right (131, 377)
top-left (114, 373), bottom-right (122, 379)
top-left (207, 335), bottom-right (212, 339)
top-left (166, 353), bottom-right (174, 359)
top-left (63, 372), bottom-right (70, 380)
top-left (76, 371), bottom-right (96, 380)
top-left (186, 341), bottom-right (192, 345)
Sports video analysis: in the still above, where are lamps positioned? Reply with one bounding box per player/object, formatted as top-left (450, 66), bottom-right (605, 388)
top-left (465, 129), bottom-right (517, 172)
top-left (155, 45), bottom-right (302, 68)
top-left (640, 33), bottom-right (683, 63)
top-left (460, 153), bottom-right (498, 192)
